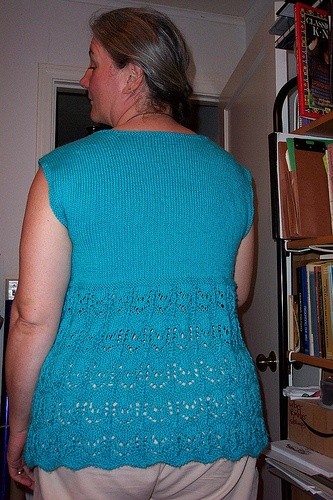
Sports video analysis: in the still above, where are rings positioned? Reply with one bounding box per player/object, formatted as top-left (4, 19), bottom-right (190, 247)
top-left (17, 468), bottom-right (25, 475)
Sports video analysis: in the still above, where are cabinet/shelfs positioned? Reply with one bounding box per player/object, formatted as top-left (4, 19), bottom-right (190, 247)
top-left (245, 0), bottom-right (332, 500)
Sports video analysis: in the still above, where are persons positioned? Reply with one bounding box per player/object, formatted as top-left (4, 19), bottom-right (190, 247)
top-left (6, 5), bottom-right (269, 499)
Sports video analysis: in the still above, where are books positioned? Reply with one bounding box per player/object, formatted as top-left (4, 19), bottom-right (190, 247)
top-left (265, 0), bottom-right (333, 499)
